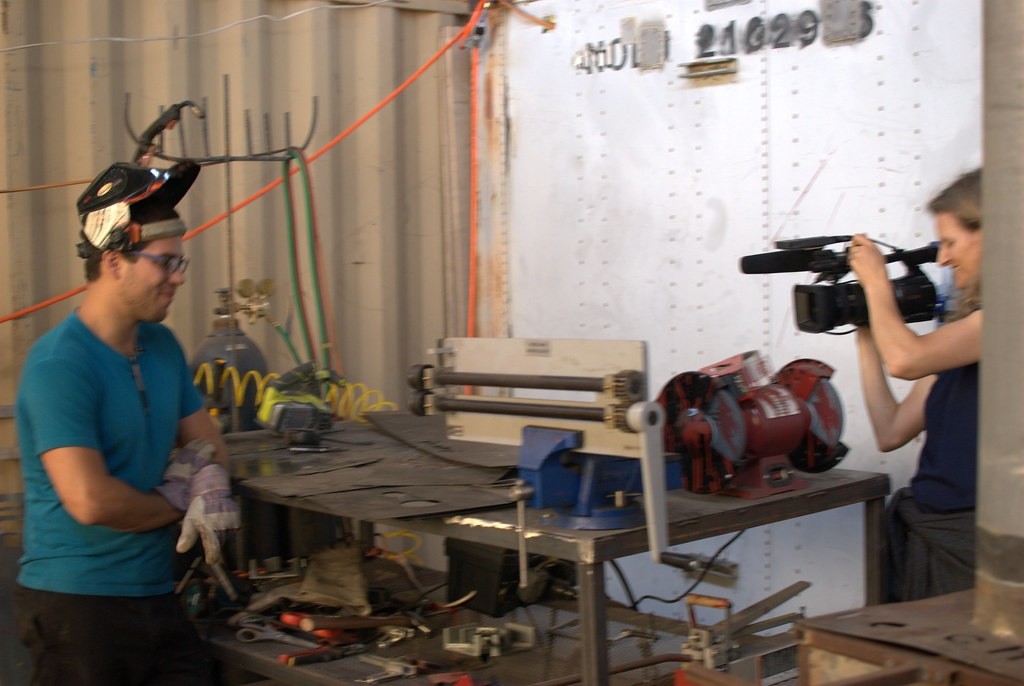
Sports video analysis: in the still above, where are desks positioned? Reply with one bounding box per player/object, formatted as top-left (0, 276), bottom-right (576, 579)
top-left (181, 468), bottom-right (891, 684)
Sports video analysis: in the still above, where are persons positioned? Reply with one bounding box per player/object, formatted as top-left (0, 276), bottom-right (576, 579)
top-left (849, 168), bottom-right (983, 602)
top-left (12, 158), bottom-right (240, 686)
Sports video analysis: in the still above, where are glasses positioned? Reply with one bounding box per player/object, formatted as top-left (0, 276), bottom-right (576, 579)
top-left (117, 248), bottom-right (189, 275)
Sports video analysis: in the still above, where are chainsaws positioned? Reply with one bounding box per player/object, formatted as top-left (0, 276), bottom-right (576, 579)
top-left (650, 350), bottom-right (851, 498)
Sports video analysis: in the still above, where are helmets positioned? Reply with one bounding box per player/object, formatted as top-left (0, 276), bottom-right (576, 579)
top-left (77, 157), bottom-right (202, 257)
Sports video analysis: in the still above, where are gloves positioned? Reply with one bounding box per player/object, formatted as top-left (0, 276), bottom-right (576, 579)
top-left (176, 463), bottom-right (242, 567)
top-left (152, 438), bottom-right (218, 514)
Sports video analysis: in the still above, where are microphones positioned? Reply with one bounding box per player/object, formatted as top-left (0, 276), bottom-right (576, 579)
top-left (741, 250), bottom-right (837, 274)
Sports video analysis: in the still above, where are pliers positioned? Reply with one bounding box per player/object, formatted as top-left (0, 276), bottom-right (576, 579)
top-left (277, 644), bottom-right (367, 667)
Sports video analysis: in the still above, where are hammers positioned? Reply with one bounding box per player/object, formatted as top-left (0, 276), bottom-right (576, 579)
top-left (300, 610), bottom-right (434, 639)
